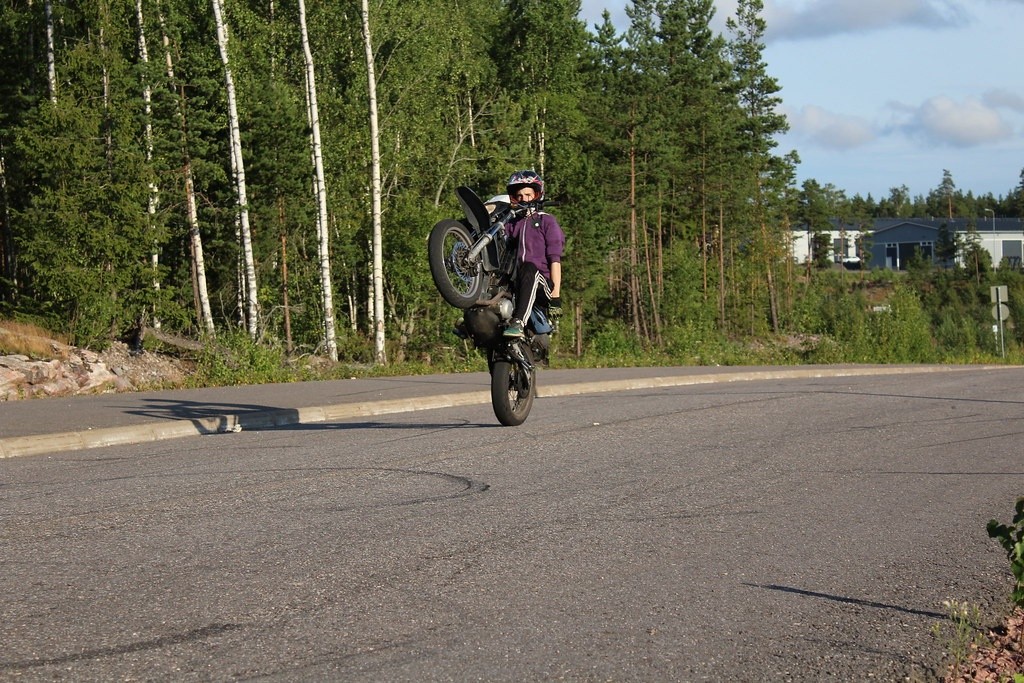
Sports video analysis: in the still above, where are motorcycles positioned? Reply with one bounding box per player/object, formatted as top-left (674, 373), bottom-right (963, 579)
top-left (424, 186), bottom-right (561, 426)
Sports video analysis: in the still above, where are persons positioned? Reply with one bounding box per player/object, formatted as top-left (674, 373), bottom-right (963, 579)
top-left (455, 170), bottom-right (566, 337)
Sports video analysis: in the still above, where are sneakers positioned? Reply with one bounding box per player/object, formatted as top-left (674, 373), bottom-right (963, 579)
top-left (502, 318), bottom-right (526, 338)
top-left (452, 328), bottom-right (468, 339)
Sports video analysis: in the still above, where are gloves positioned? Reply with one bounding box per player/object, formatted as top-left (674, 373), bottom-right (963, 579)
top-left (549, 297), bottom-right (563, 320)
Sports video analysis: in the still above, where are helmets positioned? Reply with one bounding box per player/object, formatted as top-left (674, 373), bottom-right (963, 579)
top-left (506, 171), bottom-right (544, 218)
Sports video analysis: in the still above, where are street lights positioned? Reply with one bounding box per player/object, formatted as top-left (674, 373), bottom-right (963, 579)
top-left (985, 208), bottom-right (997, 274)
